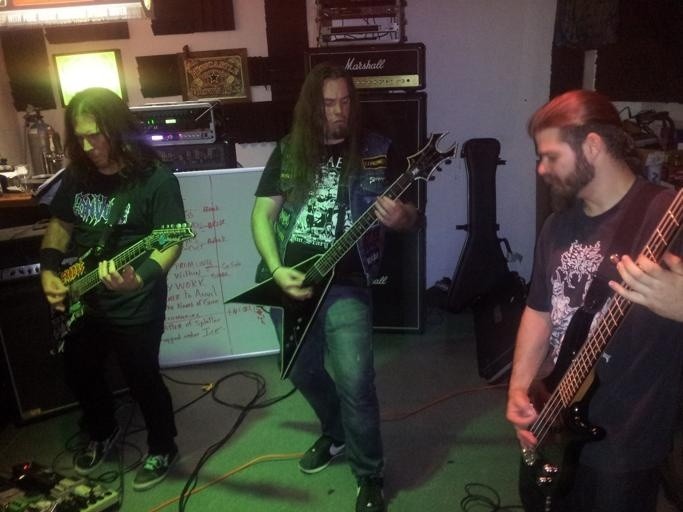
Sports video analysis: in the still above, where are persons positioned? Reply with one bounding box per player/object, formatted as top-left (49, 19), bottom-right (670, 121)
top-left (503, 88), bottom-right (682, 512)
top-left (41, 87), bottom-right (186, 490)
top-left (251, 61), bottom-right (425, 512)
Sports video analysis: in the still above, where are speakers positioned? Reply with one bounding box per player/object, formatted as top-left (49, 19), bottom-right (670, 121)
top-left (304, 43), bottom-right (427, 92)
top-left (0, 277), bottom-right (132, 427)
top-left (357, 91), bottom-right (427, 334)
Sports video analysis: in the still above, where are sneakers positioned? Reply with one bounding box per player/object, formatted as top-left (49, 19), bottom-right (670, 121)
top-left (131, 449), bottom-right (177, 491)
top-left (75, 425), bottom-right (121, 475)
top-left (355, 479), bottom-right (384, 512)
top-left (299, 436), bottom-right (346, 473)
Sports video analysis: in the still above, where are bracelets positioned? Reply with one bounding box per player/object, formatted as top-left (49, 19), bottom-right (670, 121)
top-left (270, 266), bottom-right (284, 276)
top-left (39, 248), bottom-right (68, 278)
top-left (136, 258), bottom-right (164, 289)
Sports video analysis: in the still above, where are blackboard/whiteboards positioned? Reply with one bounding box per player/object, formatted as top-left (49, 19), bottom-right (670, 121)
top-left (157, 167), bottom-right (284, 368)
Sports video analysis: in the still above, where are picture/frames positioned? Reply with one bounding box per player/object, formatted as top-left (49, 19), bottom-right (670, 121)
top-left (176, 47), bottom-right (252, 104)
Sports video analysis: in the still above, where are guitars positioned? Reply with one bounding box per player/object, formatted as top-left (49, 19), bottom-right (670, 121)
top-left (48, 220), bottom-right (196, 355)
top-left (223, 129), bottom-right (458, 380)
top-left (517, 191), bottom-right (680, 511)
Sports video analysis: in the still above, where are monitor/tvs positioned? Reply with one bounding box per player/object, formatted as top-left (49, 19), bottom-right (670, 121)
top-left (51, 48), bottom-right (130, 109)
